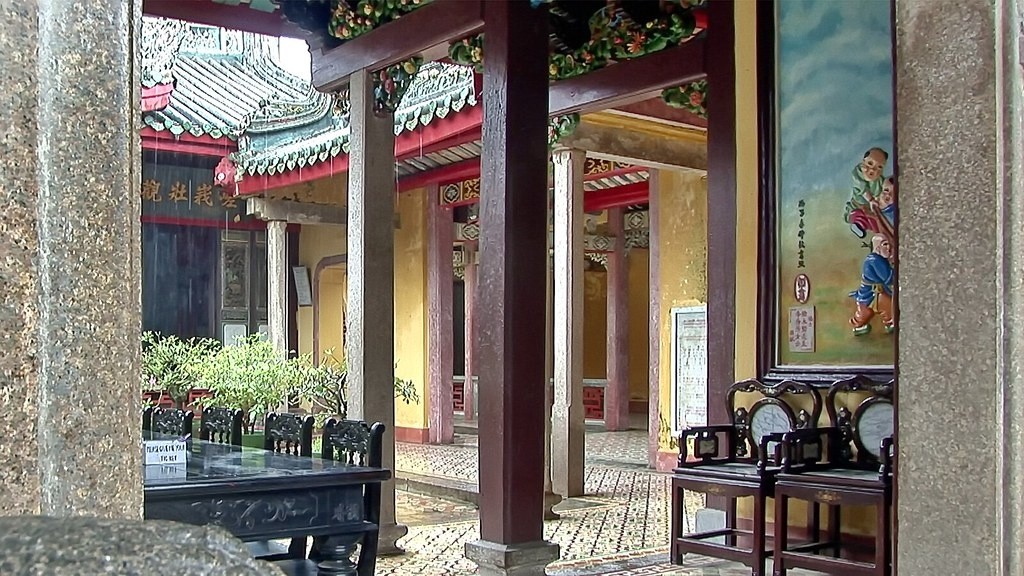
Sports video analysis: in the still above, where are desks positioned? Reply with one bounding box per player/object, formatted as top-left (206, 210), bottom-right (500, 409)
top-left (141, 432), bottom-right (390, 576)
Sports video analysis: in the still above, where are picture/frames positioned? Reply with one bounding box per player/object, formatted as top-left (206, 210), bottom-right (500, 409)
top-left (755, 1), bottom-right (898, 393)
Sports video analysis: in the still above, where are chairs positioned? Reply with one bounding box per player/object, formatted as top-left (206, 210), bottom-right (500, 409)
top-left (152, 406), bottom-right (194, 438)
top-left (201, 406), bottom-right (244, 446)
top-left (274, 419), bottom-right (385, 576)
top-left (236, 410), bottom-right (313, 561)
top-left (671, 377), bottom-right (822, 576)
top-left (772, 377), bottom-right (897, 576)
top-left (142, 404), bottom-right (153, 431)
